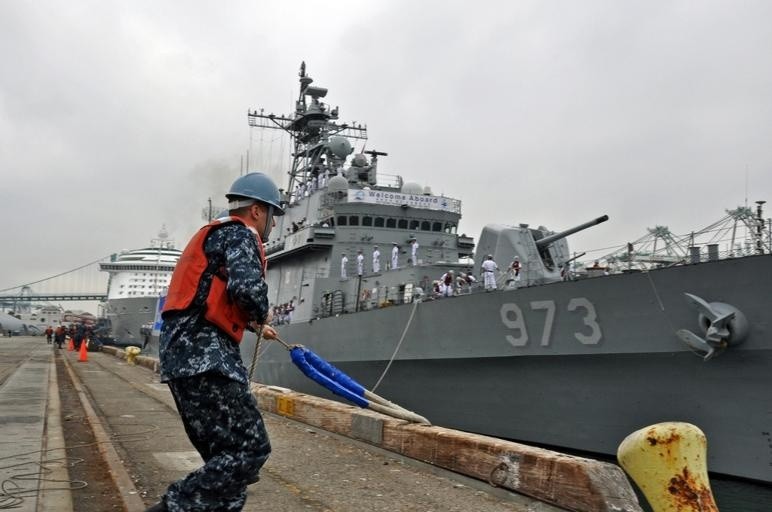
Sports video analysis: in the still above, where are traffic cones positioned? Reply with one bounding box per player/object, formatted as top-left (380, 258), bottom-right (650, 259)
top-left (67, 339), bottom-right (74, 352)
top-left (78, 340), bottom-right (88, 362)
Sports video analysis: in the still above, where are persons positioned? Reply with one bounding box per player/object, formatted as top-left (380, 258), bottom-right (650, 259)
top-left (410, 238), bottom-right (419, 265)
top-left (341, 253), bottom-right (348, 277)
top-left (372, 245), bottom-right (381, 273)
top-left (356, 250), bottom-right (364, 276)
top-left (429, 254), bottom-right (522, 299)
top-left (141, 171), bottom-right (286, 512)
top-left (44, 322), bottom-right (87, 351)
top-left (391, 242), bottom-right (399, 269)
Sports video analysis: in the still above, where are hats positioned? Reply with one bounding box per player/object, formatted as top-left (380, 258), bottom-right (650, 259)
top-left (341, 237), bottom-right (416, 255)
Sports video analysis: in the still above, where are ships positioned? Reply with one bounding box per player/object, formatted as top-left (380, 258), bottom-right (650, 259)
top-left (141, 62), bottom-right (772, 510)
top-left (1, 306), bottom-right (100, 336)
top-left (99, 223), bottom-right (184, 347)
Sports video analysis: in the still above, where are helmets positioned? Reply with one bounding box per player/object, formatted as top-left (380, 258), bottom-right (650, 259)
top-left (514, 256), bottom-right (519, 262)
top-left (225, 172), bottom-right (286, 216)
